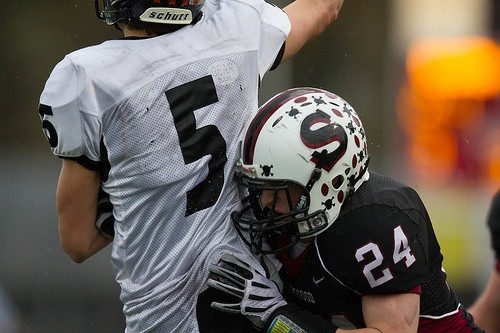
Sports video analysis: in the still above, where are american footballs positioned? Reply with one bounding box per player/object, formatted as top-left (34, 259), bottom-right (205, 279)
top-left (95, 189), bottom-right (114, 238)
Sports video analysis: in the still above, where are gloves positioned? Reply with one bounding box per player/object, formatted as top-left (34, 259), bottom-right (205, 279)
top-left (206, 253), bottom-right (289, 327)
top-left (95, 193), bottom-right (114, 239)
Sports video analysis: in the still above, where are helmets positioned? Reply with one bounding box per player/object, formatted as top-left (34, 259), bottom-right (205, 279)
top-left (104, 0), bottom-right (203, 26)
top-left (234, 87), bottom-right (371, 239)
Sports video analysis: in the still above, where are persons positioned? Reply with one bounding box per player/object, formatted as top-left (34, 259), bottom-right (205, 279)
top-left (209, 87), bottom-right (484, 333)
top-left (468, 190), bottom-right (500, 333)
top-left (39, 0), bottom-right (346, 333)
